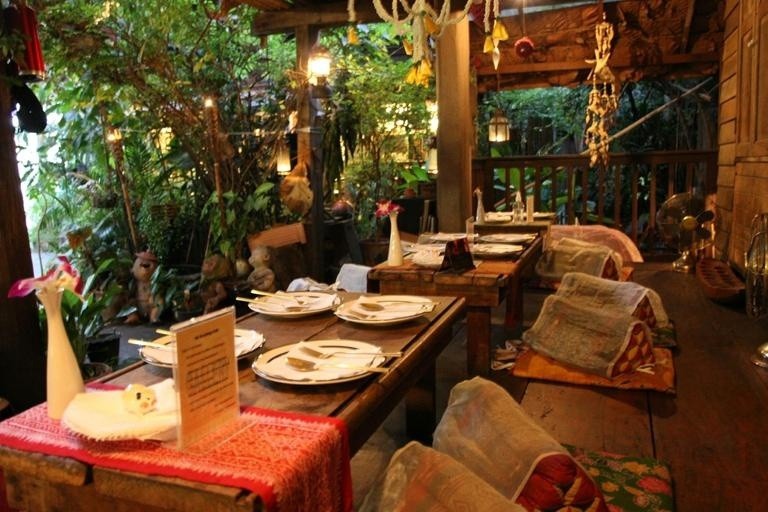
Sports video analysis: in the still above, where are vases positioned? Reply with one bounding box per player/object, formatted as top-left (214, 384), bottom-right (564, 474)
top-left (387, 213), bottom-right (403, 265)
top-left (33, 293), bottom-right (87, 420)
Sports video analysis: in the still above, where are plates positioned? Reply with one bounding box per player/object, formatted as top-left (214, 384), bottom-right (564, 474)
top-left (247, 293), bottom-right (341, 318)
top-left (412, 232), bottom-right (535, 268)
top-left (139, 327), bottom-right (263, 368)
top-left (338, 293), bottom-right (434, 326)
top-left (252, 339), bottom-right (385, 384)
top-left (482, 210), bottom-right (555, 225)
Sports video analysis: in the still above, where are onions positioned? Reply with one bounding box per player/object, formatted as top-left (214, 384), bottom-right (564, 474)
top-left (332, 199), bottom-right (353, 216)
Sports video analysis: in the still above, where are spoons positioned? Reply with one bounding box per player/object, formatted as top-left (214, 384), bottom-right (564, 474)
top-left (359, 302), bottom-right (442, 312)
top-left (287, 358), bottom-right (391, 374)
top-left (234, 296), bottom-right (310, 312)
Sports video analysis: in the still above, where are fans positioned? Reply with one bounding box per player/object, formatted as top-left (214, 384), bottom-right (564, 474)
top-left (654, 191), bottom-right (722, 275)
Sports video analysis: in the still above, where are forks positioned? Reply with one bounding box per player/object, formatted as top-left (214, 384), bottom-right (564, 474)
top-left (297, 341), bottom-right (403, 359)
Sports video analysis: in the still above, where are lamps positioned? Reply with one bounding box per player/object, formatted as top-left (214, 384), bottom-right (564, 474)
top-left (486, 65), bottom-right (512, 142)
top-left (307, 0), bottom-right (332, 78)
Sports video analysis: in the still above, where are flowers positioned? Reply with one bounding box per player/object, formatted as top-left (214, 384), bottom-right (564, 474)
top-left (5, 253), bottom-right (87, 305)
top-left (374, 198), bottom-right (405, 217)
